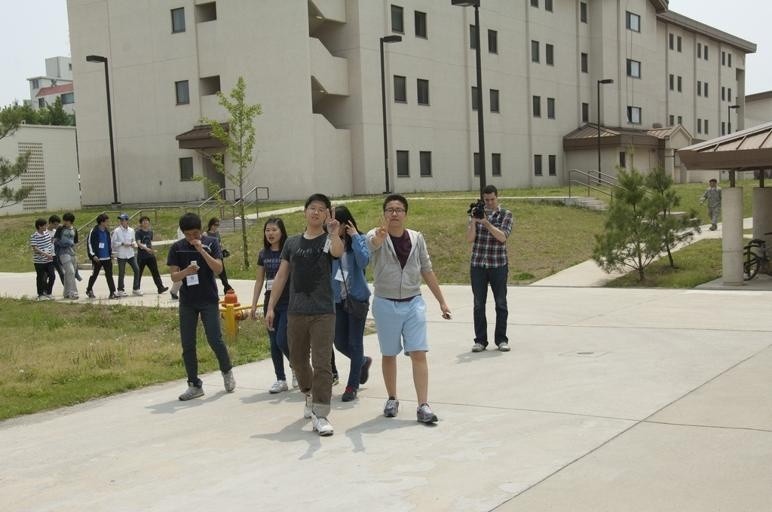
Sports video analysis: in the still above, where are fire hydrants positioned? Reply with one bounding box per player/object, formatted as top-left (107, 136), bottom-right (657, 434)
top-left (221, 289), bottom-right (249, 339)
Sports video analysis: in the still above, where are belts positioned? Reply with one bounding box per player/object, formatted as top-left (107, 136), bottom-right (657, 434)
top-left (384, 294), bottom-right (421, 302)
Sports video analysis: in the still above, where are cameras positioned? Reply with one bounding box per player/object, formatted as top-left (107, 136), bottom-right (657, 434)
top-left (467, 199), bottom-right (485, 219)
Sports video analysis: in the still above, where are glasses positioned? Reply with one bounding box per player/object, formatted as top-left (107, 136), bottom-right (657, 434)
top-left (384, 208), bottom-right (406, 214)
top-left (213, 224), bottom-right (220, 226)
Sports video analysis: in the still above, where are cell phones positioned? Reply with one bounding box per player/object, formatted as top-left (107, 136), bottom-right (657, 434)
top-left (191, 261), bottom-right (196, 265)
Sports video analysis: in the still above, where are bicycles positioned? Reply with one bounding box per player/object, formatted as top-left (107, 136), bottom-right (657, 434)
top-left (743, 231), bottom-right (772, 281)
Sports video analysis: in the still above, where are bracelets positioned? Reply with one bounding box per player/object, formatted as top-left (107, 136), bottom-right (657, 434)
top-left (201, 251), bottom-right (208, 256)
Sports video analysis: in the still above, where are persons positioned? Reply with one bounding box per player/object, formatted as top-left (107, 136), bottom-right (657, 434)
top-left (304, 209), bottom-right (340, 387)
top-left (251, 218), bottom-right (299, 394)
top-left (30, 211), bottom-right (234, 303)
top-left (264, 197), bottom-right (346, 436)
top-left (698, 178), bottom-right (721, 231)
top-left (330, 206), bottom-right (372, 402)
top-left (366, 195), bottom-right (453, 424)
top-left (166, 213), bottom-right (236, 400)
top-left (466, 185), bottom-right (513, 351)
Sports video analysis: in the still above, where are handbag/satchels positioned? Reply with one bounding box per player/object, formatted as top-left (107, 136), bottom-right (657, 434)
top-left (222, 250), bottom-right (230, 257)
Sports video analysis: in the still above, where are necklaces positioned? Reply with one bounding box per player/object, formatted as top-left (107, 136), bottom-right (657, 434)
top-left (306, 228), bottom-right (324, 236)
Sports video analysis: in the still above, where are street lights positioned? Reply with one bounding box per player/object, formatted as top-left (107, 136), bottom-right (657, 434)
top-left (85, 54), bottom-right (123, 205)
top-left (727, 104), bottom-right (740, 134)
top-left (380, 34), bottom-right (404, 195)
top-left (597, 78), bottom-right (614, 186)
top-left (450, 0), bottom-right (487, 200)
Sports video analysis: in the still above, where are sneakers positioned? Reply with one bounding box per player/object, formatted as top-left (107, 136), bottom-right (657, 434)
top-left (179, 356), bottom-right (438, 435)
top-left (37, 276), bottom-right (180, 301)
top-left (497, 342), bottom-right (510, 351)
top-left (472, 343), bottom-right (486, 352)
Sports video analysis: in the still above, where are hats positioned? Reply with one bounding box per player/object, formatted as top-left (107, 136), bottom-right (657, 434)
top-left (117, 215), bottom-right (128, 220)
